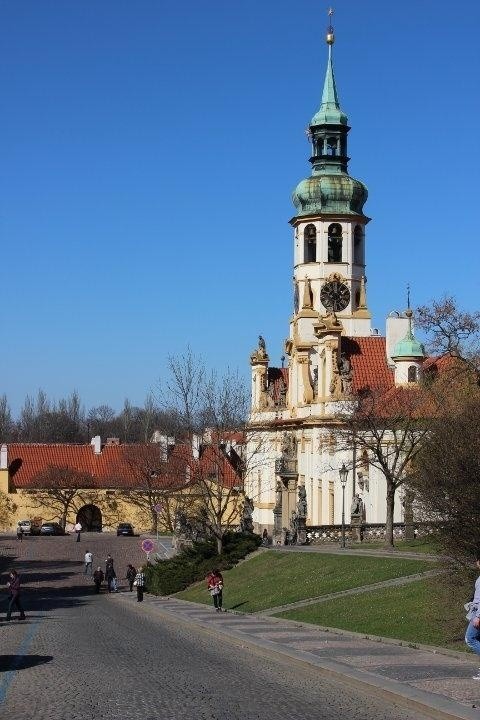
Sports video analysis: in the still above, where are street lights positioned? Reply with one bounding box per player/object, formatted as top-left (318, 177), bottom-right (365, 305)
top-left (338, 463), bottom-right (349, 548)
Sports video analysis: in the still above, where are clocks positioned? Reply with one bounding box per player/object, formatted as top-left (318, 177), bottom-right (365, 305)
top-left (320, 282), bottom-right (350, 311)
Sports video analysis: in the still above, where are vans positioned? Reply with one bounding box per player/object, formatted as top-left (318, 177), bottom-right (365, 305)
top-left (17, 520), bottom-right (40, 536)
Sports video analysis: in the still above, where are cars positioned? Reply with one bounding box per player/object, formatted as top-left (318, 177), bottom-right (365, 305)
top-left (117, 522), bottom-right (135, 537)
top-left (41, 521), bottom-right (64, 536)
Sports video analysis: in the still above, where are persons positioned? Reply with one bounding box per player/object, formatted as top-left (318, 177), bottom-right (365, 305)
top-left (74, 521), bottom-right (83, 542)
top-left (84, 549), bottom-right (147, 604)
top-left (263, 529), bottom-right (269, 546)
top-left (207, 568), bottom-right (225, 613)
top-left (16, 523), bottom-right (25, 542)
top-left (465, 549), bottom-right (480, 680)
top-left (5, 569), bottom-right (25, 623)
